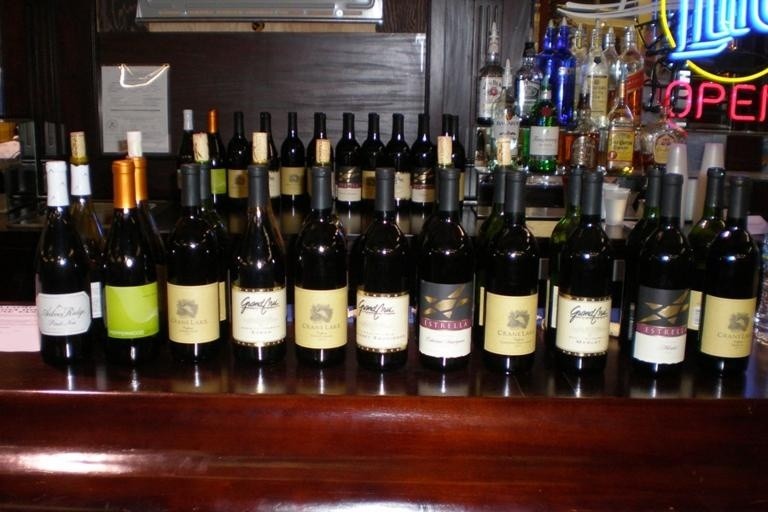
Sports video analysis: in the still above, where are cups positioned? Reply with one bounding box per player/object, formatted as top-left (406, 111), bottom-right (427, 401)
top-left (753, 237), bottom-right (768, 345)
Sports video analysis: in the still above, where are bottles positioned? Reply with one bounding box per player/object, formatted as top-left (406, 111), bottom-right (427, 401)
top-left (471, 18), bottom-right (687, 172)
top-left (36, 110), bottom-right (762, 380)
